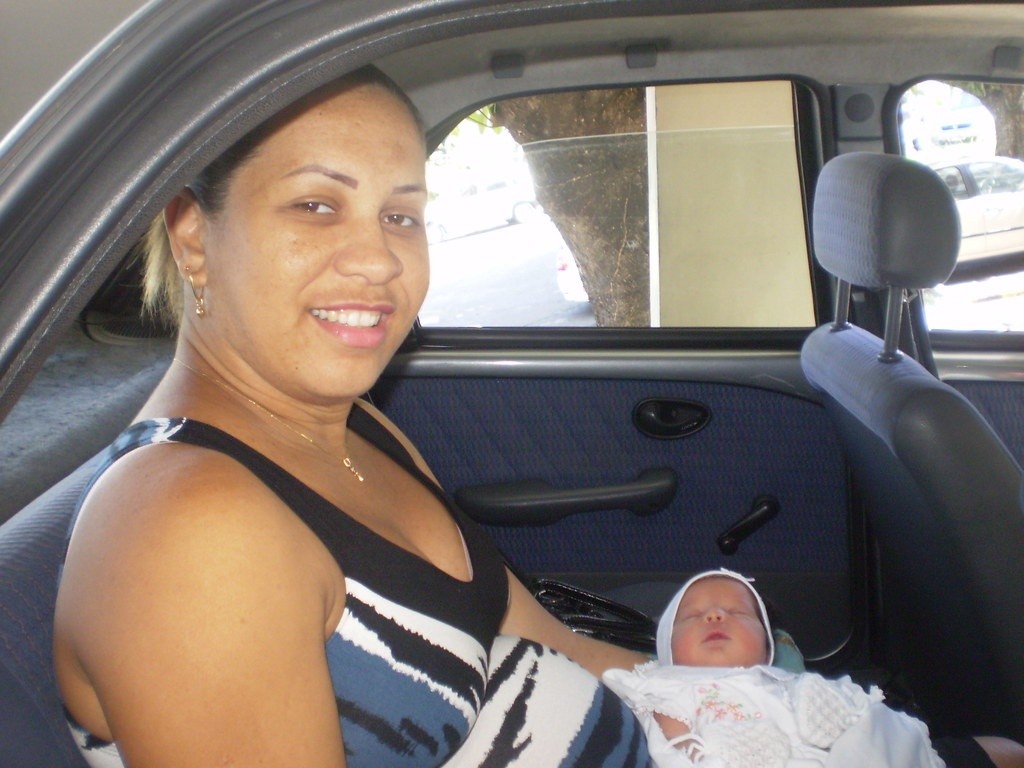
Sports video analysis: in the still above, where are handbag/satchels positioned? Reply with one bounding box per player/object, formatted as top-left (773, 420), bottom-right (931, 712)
top-left (530, 577), bottom-right (658, 649)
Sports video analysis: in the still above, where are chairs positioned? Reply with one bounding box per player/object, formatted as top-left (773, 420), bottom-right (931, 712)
top-left (802, 154), bottom-right (1024, 729)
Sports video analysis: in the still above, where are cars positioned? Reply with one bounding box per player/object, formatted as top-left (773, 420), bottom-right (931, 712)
top-left (934, 156), bottom-right (1024, 275)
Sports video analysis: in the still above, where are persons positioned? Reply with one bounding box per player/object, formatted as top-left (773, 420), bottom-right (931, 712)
top-left (53, 63), bottom-right (1024, 768)
top-left (607, 567), bottom-right (948, 768)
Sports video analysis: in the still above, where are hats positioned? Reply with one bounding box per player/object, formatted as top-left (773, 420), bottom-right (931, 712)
top-left (657, 568), bottom-right (775, 665)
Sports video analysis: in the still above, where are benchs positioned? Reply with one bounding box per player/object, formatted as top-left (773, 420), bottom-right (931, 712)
top-left (0, 339), bottom-right (167, 768)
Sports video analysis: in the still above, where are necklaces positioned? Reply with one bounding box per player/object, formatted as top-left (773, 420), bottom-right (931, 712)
top-left (173, 358), bottom-right (363, 482)
